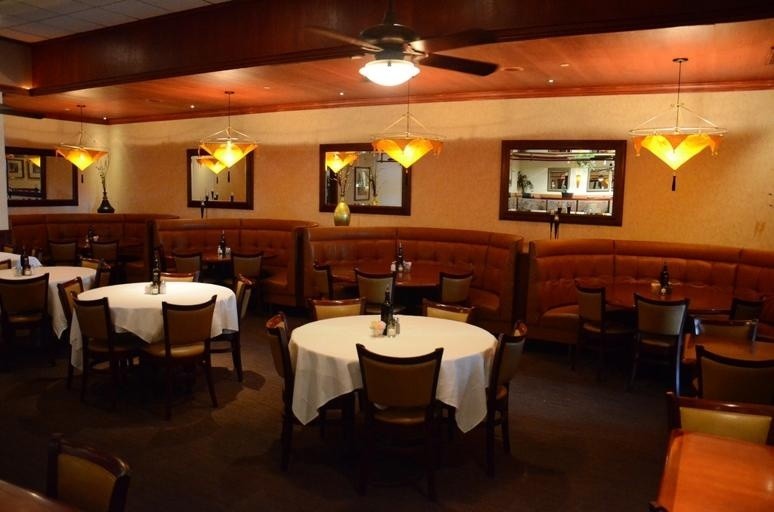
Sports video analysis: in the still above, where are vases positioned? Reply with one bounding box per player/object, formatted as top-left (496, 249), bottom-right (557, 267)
top-left (334, 194), bottom-right (350, 226)
top-left (97, 192), bottom-right (114, 213)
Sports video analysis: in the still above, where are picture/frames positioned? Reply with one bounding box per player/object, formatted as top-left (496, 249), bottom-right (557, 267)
top-left (354, 167), bottom-right (370, 201)
top-left (547, 168), bottom-right (571, 191)
top-left (587, 168), bottom-right (611, 191)
top-left (7, 159), bottom-right (24, 177)
top-left (28, 160), bottom-right (41, 179)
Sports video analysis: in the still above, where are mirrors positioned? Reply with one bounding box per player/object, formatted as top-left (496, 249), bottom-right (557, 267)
top-left (319, 142), bottom-right (412, 215)
top-left (498, 137), bottom-right (628, 226)
top-left (187, 148), bottom-right (255, 209)
top-left (6, 146), bottom-right (77, 206)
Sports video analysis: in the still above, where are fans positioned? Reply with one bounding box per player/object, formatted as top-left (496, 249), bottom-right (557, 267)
top-left (302, 1), bottom-right (501, 96)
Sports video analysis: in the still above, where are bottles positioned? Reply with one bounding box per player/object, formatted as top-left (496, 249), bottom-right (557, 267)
top-left (659, 264), bottom-right (673, 294)
top-left (14, 245), bottom-right (32, 276)
top-left (217, 229), bottom-right (232, 255)
top-left (381, 290), bottom-right (400, 336)
top-left (390, 242), bottom-right (404, 272)
top-left (149, 251), bottom-right (167, 295)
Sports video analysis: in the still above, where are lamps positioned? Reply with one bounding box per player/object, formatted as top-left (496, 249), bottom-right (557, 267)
top-left (325, 151), bottom-right (361, 173)
top-left (197, 159), bottom-right (230, 175)
top-left (368, 81), bottom-right (445, 169)
top-left (54, 105), bottom-right (109, 183)
top-left (197, 91), bottom-right (259, 172)
top-left (627, 57), bottom-right (728, 191)
top-left (29, 157), bottom-right (43, 167)
top-left (348, 55), bottom-right (423, 90)
